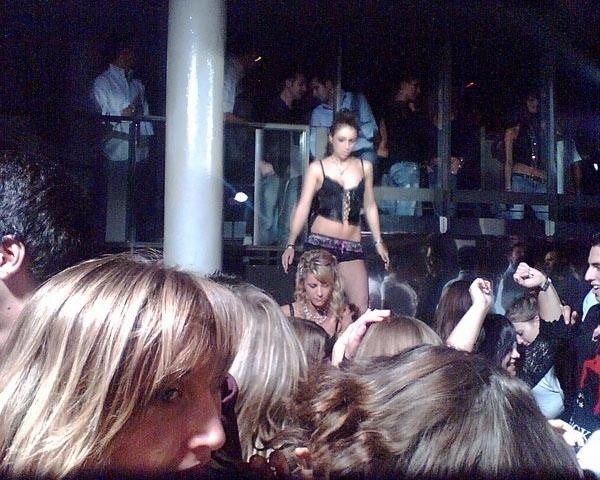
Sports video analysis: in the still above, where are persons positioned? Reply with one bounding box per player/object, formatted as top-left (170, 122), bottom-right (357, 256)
top-left (0, 35), bottom-right (599, 479)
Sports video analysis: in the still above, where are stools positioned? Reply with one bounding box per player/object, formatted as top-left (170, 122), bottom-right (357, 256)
top-left (512, 173), bottom-right (547, 184)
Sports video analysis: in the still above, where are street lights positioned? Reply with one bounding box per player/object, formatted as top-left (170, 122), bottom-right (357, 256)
top-left (491, 131), bottom-right (506, 163)
